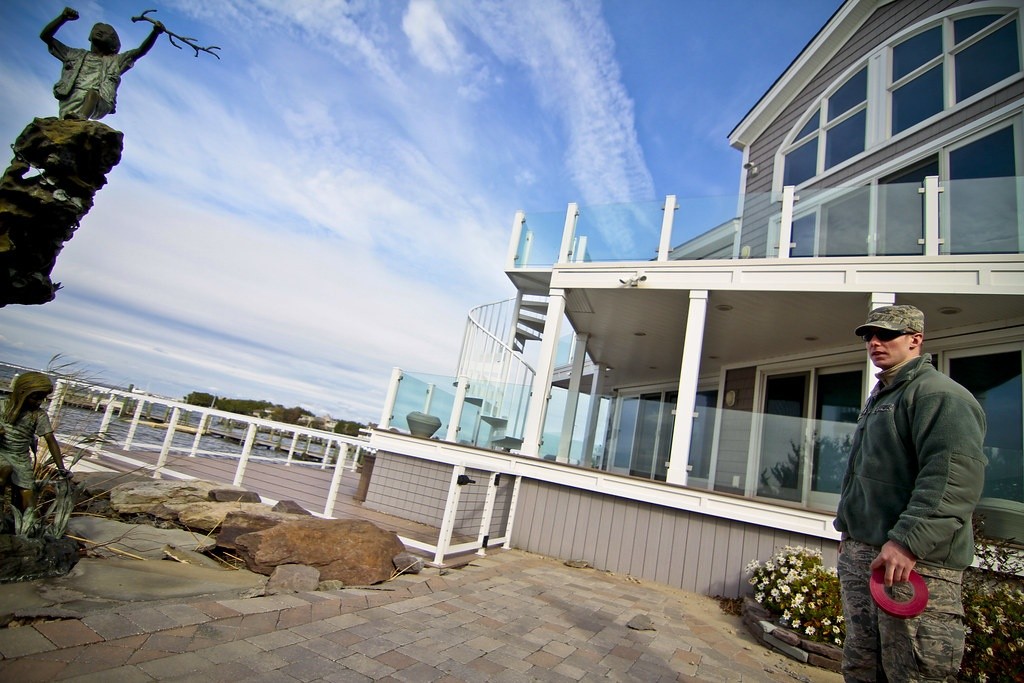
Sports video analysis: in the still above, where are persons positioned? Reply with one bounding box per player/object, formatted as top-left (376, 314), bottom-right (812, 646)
top-left (0, 371), bottom-right (74, 519)
top-left (40, 8), bottom-right (165, 121)
top-left (833, 305), bottom-right (986, 683)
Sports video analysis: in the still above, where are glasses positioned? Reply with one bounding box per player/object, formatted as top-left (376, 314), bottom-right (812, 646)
top-left (862, 328), bottom-right (916, 342)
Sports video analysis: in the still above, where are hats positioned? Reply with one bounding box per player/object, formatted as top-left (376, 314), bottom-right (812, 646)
top-left (854, 305), bottom-right (925, 337)
